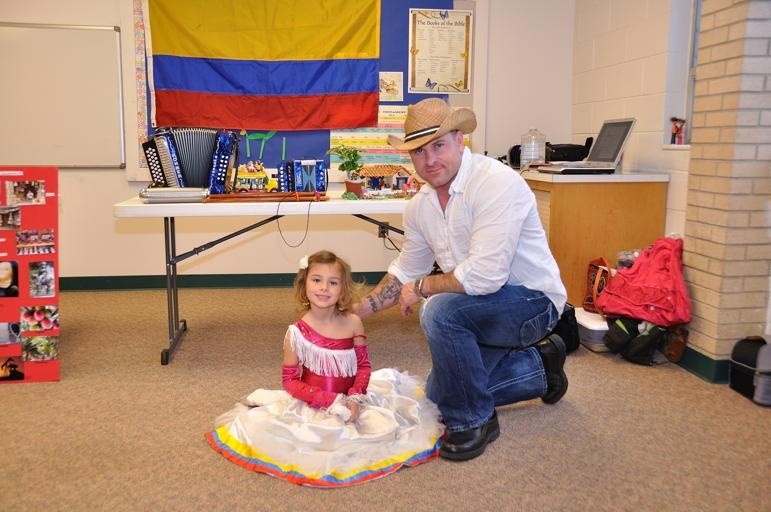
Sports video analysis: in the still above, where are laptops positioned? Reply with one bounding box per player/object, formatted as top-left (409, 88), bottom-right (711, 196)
top-left (536, 117), bottom-right (636, 175)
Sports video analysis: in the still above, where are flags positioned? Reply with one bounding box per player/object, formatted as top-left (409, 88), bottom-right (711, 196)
top-left (141, 0), bottom-right (382, 129)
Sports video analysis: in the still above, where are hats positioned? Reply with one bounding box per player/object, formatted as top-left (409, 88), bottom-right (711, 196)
top-left (388, 98), bottom-right (477, 151)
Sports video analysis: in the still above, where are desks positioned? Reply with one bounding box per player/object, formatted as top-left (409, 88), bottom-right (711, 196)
top-left (112, 194), bottom-right (406, 366)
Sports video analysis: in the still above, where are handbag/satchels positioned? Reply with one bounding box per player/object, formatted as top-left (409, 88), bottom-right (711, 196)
top-left (583, 257), bottom-right (617, 314)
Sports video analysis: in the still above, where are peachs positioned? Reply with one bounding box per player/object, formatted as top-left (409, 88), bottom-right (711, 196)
top-left (23, 309), bottom-right (58, 330)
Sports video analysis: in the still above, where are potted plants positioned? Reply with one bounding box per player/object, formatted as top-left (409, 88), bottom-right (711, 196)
top-left (322, 144), bottom-right (364, 197)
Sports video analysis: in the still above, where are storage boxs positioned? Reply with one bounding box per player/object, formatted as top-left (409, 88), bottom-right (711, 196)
top-left (574, 307), bottom-right (610, 354)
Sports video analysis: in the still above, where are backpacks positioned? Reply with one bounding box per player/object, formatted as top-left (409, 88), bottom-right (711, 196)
top-left (728, 335), bottom-right (771, 406)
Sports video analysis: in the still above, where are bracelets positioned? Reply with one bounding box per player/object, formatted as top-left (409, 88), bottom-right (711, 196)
top-left (415, 277), bottom-right (429, 298)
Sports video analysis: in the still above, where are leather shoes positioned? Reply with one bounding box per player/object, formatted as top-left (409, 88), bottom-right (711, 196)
top-left (536, 334), bottom-right (568, 404)
top-left (440, 412), bottom-right (500, 462)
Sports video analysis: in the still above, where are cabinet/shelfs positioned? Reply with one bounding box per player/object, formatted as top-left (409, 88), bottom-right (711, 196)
top-left (506, 170), bottom-right (670, 309)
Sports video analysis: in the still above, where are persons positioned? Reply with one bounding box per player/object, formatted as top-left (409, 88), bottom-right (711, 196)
top-left (0, 177), bottom-right (60, 380)
top-left (208, 247), bottom-right (448, 489)
top-left (346, 93), bottom-right (570, 461)
